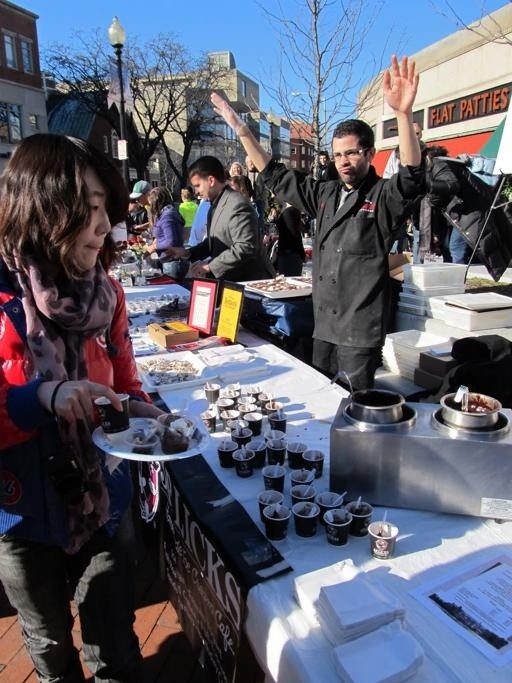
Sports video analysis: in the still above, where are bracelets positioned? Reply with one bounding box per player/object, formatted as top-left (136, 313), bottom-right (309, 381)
top-left (51, 379), bottom-right (67, 415)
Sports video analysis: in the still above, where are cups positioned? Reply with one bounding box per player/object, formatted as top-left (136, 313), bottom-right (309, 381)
top-left (122, 430), bottom-right (161, 454)
top-left (200, 383), bottom-right (375, 547)
top-left (368, 520), bottom-right (399, 560)
top-left (129, 418), bottom-right (160, 432)
top-left (94, 393), bottom-right (130, 433)
top-left (156, 413), bottom-right (192, 455)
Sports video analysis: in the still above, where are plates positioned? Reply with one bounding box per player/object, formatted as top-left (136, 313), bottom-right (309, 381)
top-left (91, 417), bottom-right (210, 462)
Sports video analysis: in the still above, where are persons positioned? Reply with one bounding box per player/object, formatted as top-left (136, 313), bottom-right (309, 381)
top-left (310, 151), bottom-right (330, 181)
top-left (124, 154), bottom-right (305, 339)
top-left (382, 122), bottom-right (510, 280)
top-left (211, 55), bottom-right (420, 393)
top-left (0, 133), bottom-right (155, 683)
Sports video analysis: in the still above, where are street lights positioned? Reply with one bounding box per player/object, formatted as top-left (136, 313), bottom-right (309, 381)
top-left (108, 14), bottom-right (131, 194)
top-left (265, 107), bottom-right (275, 158)
top-left (290, 91), bottom-right (326, 151)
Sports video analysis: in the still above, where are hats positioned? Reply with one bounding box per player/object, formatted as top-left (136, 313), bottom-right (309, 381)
top-left (128, 179), bottom-right (154, 200)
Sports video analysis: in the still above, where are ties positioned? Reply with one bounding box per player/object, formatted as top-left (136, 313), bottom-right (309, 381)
top-left (334, 186), bottom-right (356, 217)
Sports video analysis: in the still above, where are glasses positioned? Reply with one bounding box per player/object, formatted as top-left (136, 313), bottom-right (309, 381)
top-left (329, 145), bottom-right (370, 163)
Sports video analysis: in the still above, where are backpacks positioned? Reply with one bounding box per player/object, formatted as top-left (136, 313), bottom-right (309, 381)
top-left (433, 152), bottom-right (503, 198)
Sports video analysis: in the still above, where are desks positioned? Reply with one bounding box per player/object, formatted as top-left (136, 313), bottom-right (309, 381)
top-left (102, 218), bottom-right (512, 683)
